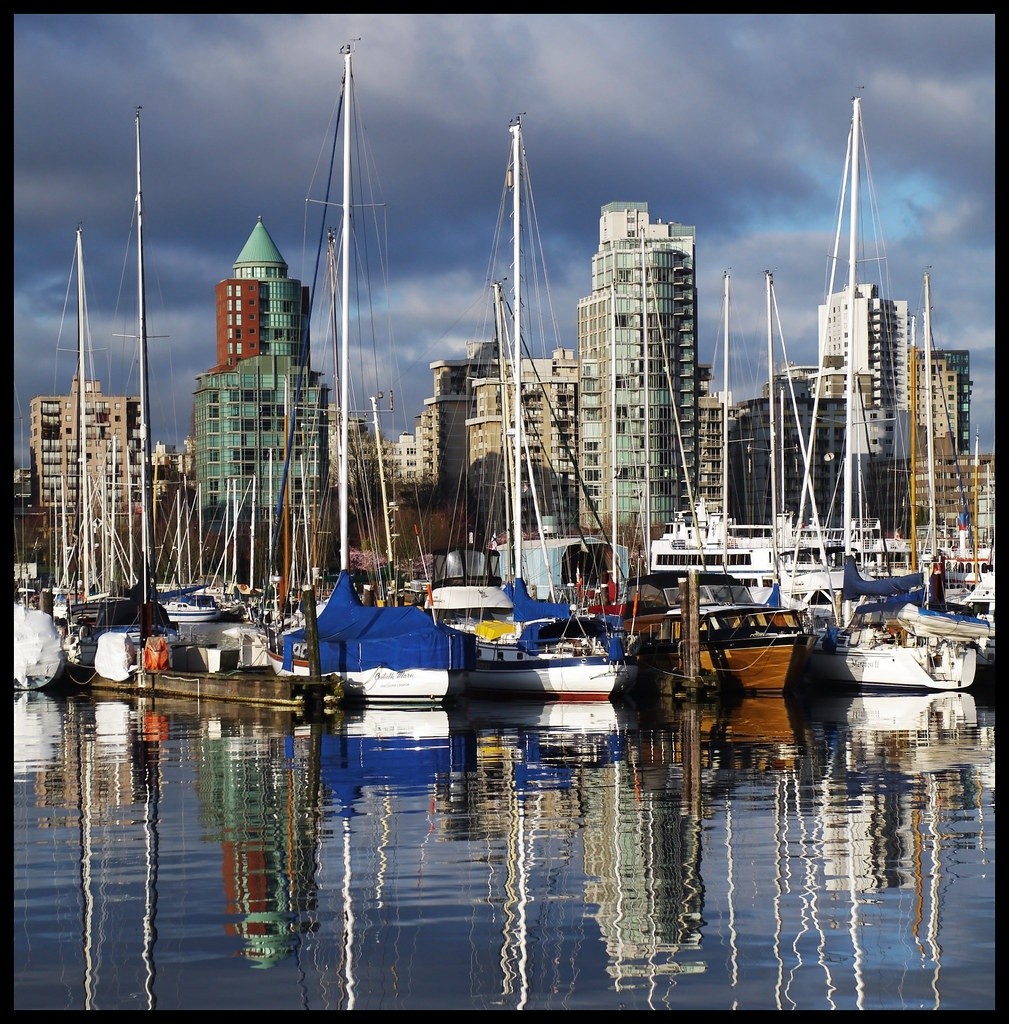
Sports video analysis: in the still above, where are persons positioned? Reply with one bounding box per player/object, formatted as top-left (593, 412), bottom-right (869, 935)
top-left (827, 554), bottom-right (832, 566)
top-left (385, 579), bottom-right (396, 606)
top-left (706, 588), bottom-right (720, 602)
top-left (894, 527), bottom-right (902, 542)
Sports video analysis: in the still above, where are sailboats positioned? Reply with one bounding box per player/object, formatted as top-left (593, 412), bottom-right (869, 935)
top-left (15, 47), bottom-right (993, 710)
